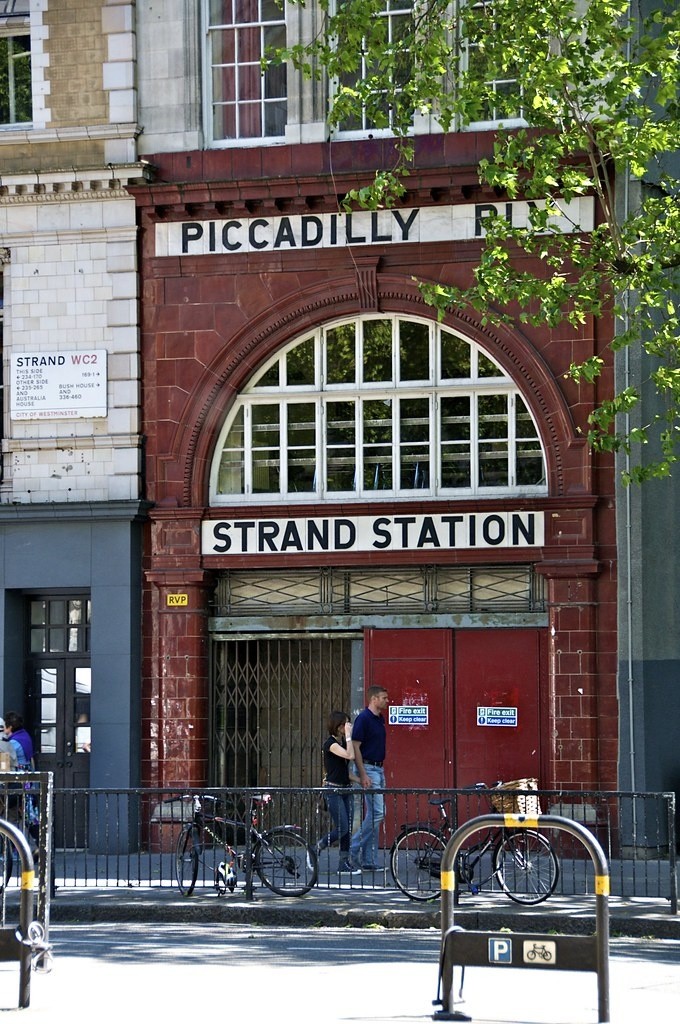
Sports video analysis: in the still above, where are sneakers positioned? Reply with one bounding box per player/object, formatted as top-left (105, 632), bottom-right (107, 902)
top-left (351, 852), bottom-right (360, 868)
top-left (362, 862), bottom-right (390, 871)
top-left (306, 843), bottom-right (320, 872)
top-left (336, 860), bottom-right (361, 875)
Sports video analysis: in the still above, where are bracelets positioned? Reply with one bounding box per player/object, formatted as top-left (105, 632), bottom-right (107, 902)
top-left (346, 737), bottom-right (351, 742)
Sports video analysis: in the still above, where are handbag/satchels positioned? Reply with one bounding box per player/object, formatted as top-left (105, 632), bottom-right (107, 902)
top-left (318, 794), bottom-right (327, 812)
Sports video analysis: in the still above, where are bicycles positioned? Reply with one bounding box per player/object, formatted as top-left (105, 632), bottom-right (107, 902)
top-left (387, 777), bottom-right (561, 906)
top-left (164, 793), bottom-right (320, 896)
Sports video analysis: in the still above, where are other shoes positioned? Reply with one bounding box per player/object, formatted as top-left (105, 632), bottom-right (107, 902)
top-left (1, 852), bottom-right (22, 861)
top-left (32, 849), bottom-right (39, 865)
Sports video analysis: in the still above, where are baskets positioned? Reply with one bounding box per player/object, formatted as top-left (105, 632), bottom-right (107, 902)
top-left (488, 779), bottom-right (542, 817)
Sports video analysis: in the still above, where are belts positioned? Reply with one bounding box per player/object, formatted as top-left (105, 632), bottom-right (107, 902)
top-left (324, 781), bottom-right (352, 788)
top-left (362, 759), bottom-right (384, 768)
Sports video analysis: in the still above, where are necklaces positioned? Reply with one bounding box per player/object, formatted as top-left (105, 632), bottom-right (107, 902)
top-left (368, 707), bottom-right (376, 715)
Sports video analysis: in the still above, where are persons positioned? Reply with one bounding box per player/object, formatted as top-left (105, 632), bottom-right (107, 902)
top-left (0, 711), bottom-right (40, 864)
top-left (351, 687), bottom-right (389, 872)
top-left (306, 712), bottom-right (372, 876)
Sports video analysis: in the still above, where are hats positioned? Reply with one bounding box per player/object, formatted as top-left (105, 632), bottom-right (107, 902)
top-left (0, 718), bottom-right (5, 731)
